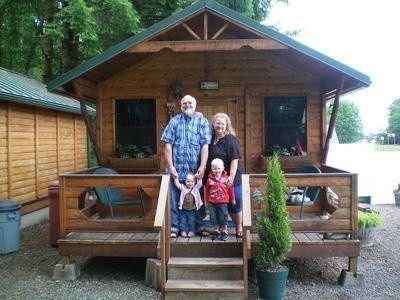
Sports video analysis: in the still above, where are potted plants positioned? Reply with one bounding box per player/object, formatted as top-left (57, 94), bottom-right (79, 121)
top-left (358, 210), bottom-right (383, 249)
top-left (253, 151), bottom-right (292, 300)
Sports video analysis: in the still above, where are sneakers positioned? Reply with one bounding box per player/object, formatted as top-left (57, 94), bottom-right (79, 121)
top-left (171, 229), bottom-right (229, 241)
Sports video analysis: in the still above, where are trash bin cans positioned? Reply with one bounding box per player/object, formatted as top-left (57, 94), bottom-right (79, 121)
top-left (0, 200), bottom-right (21, 255)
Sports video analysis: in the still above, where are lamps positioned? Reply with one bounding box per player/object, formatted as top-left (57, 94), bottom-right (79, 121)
top-left (170, 80), bottom-right (184, 100)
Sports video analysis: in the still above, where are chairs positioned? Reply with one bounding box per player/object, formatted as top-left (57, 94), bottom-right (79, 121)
top-left (285, 163), bottom-right (323, 219)
top-left (90, 166), bottom-right (148, 219)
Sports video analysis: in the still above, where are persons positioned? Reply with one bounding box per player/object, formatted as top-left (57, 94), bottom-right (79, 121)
top-left (159, 96), bottom-right (212, 238)
top-left (206, 158), bottom-right (235, 241)
top-left (172, 172), bottom-right (204, 238)
top-left (195, 111), bottom-right (245, 238)
